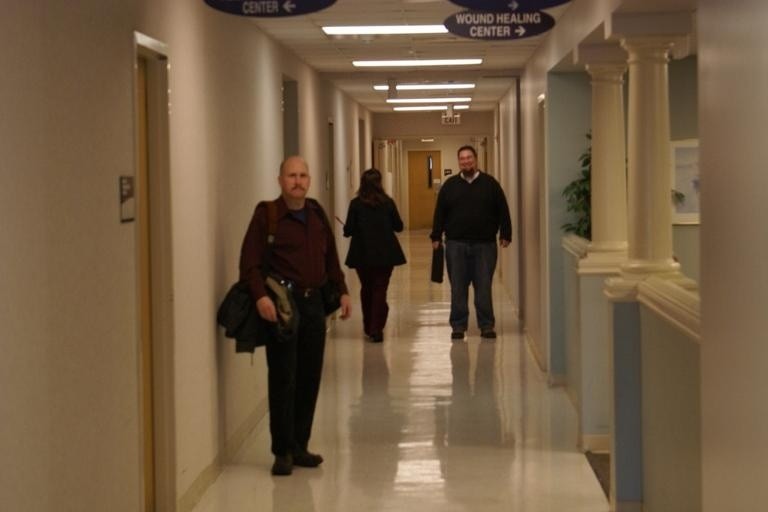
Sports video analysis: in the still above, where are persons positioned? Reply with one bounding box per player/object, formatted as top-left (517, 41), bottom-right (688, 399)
top-left (238, 154), bottom-right (353, 475)
top-left (348, 339), bottom-right (412, 500)
top-left (268, 467), bottom-right (324, 511)
top-left (429, 144), bottom-right (513, 339)
top-left (342, 168), bottom-right (408, 343)
top-left (432, 336), bottom-right (517, 510)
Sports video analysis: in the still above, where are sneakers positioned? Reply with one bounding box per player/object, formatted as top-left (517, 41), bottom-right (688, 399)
top-left (271, 449), bottom-right (295, 475)
top-left (482, 328), bottom-right (496, 337)
top-left (363, 329), bottom-right (383, 342)
top-left (293, 452), bottom-right (322, 467)
top-left (452, 329), bottom-right (464, 339)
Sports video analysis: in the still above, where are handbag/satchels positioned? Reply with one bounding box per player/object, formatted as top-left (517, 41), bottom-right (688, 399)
top-left (262, 274), bottom-right (299, 344)
top-left (322, 281), bottom-right (341, 316)
top-left (431, 246), bottom-right (444, 284)
